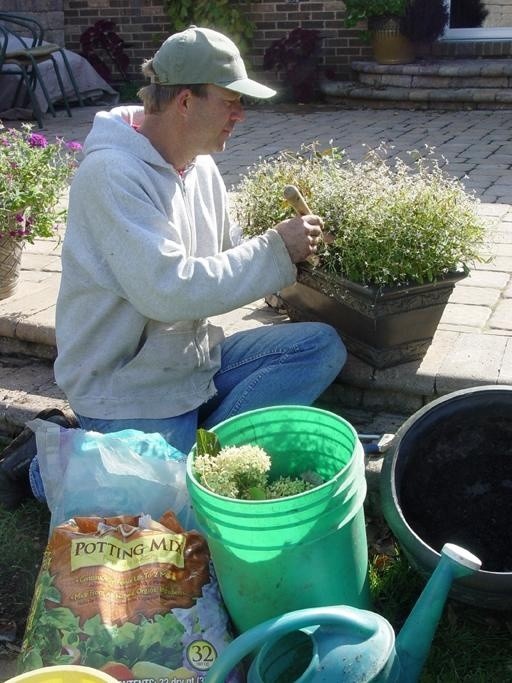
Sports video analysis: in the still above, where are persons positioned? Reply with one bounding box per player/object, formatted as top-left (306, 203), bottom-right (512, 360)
top-left (0, 26), bottom-right (349, 510)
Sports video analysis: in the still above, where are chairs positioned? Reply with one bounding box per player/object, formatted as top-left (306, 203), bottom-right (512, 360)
top-left (0, 12), bottom-right (86, 128)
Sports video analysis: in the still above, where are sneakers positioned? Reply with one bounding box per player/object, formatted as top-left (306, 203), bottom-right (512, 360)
top-left (0, 407), bottom-right (71, 511)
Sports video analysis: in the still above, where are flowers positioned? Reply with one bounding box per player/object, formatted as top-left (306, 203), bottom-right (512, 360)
top-left (190, 442), bottom-right (327, 500)
top-left (0, 119), bottom-right (86, 246)
top-left (225, 138), bottom-right (508, 309)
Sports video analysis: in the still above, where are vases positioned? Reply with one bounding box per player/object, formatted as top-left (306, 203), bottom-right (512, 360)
top-left (263, 262), bottom-right (470, 372)
top-left (0, 205), bottom-right (32, 302)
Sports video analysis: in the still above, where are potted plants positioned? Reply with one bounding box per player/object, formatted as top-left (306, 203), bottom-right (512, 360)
top-left (342, 1), bottom-right (450, 64)
top-left (262, 27), bottom-right (323, 104)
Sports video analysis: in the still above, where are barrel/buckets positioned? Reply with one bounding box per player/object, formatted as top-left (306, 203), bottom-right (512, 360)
top-left (5, 664), bottom-right (119, 683)
top-left (381, 384), bottom-right (511, 609)
top-left (186, 404), bottom-right (376, 637)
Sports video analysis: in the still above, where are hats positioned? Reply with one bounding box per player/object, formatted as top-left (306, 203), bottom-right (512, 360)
top-left (151, 24), bottom-right (277, 98)
